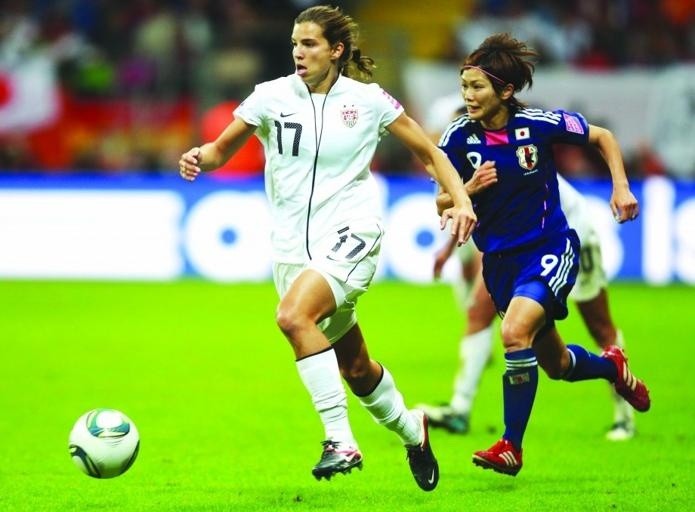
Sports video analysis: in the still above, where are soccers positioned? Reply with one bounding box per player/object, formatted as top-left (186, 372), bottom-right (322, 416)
top-left (70, 409), bottom-right (140, 478)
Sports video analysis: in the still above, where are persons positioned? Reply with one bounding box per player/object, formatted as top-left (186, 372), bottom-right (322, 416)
top-left (178, 5), bottom-right (477, 491)
top-left (414, 106), bottom-right (634, 443)
top-left (434, 34), bottom-right (650, 476)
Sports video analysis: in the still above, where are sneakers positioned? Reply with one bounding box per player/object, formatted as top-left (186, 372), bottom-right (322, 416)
top-left (600, 344), bottom-right (650, 412)
top-left (312, 440), bottom-right (363, 481)
top-left (403, 409), bottom-right (439, 491)
top-left (605, 403), bottom-right (635, 441)
top-left (472, 438), bottom-right (522, 476)
top-left (419, 404), bottom-right (471, 434)
